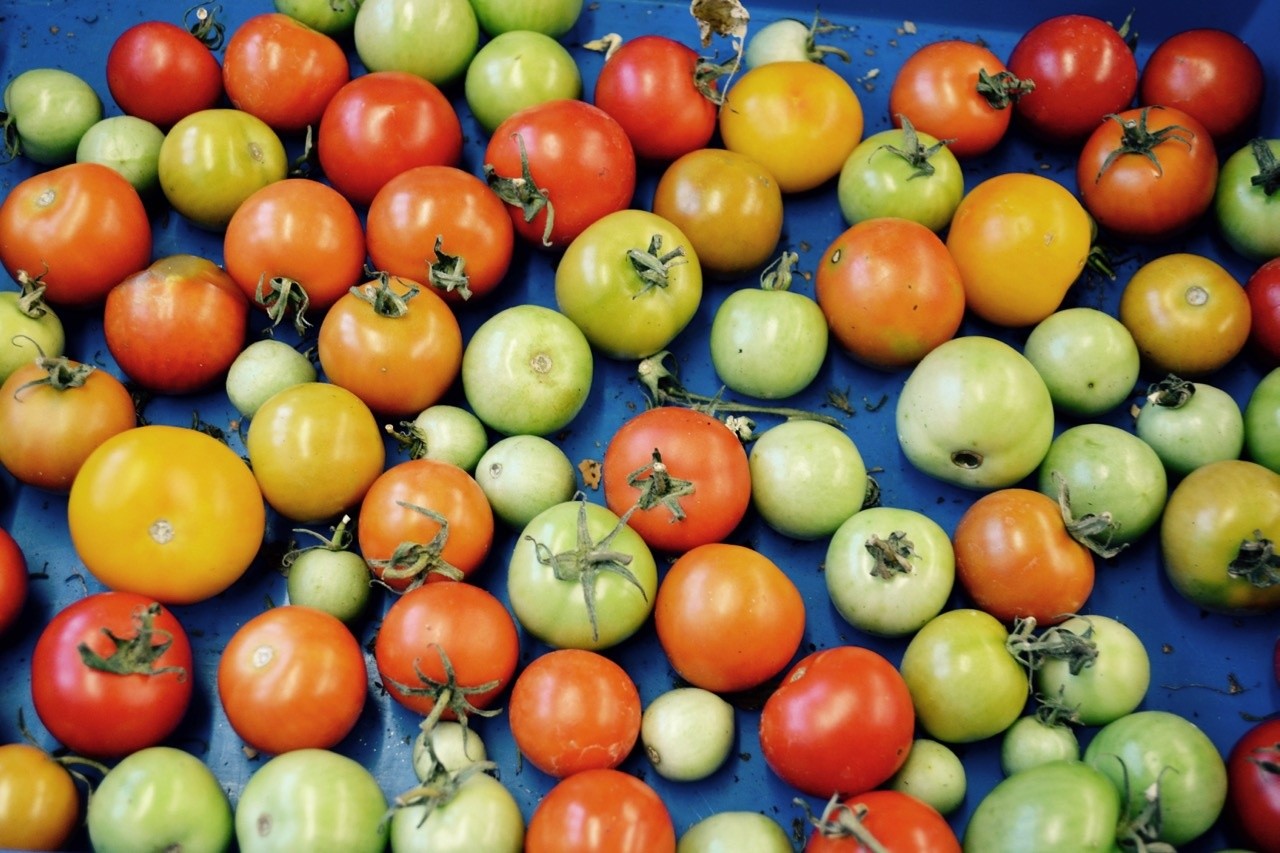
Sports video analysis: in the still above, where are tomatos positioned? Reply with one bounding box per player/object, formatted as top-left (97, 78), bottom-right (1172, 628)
top-left (0, 0), bottom-right (1280, 853)
